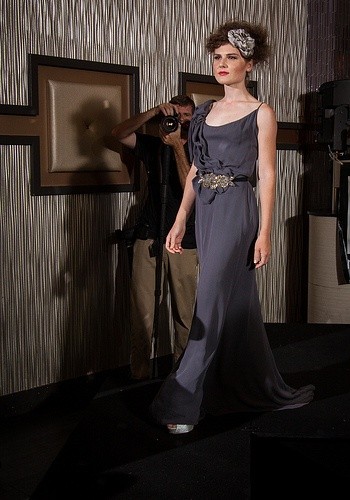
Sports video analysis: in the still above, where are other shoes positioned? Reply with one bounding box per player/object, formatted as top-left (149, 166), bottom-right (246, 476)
top-left (167, 423), bottom-right (194, 434)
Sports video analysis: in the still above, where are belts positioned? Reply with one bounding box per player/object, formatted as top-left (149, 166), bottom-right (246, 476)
top-left (197, 171), bottom-right (250, 190)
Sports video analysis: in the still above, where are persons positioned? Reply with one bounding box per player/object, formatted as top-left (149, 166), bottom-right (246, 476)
top-left (107, 96), bottom-right (220, 391)
top-left (150, 21), bottom-right (316, 435)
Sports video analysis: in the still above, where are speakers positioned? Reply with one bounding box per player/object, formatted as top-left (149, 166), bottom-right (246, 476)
top-left (320, 79), bottom-right (350, 152)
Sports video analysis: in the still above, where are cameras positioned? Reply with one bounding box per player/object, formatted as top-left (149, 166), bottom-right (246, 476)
top-left (161, 108), bottom-right (179, 134)
